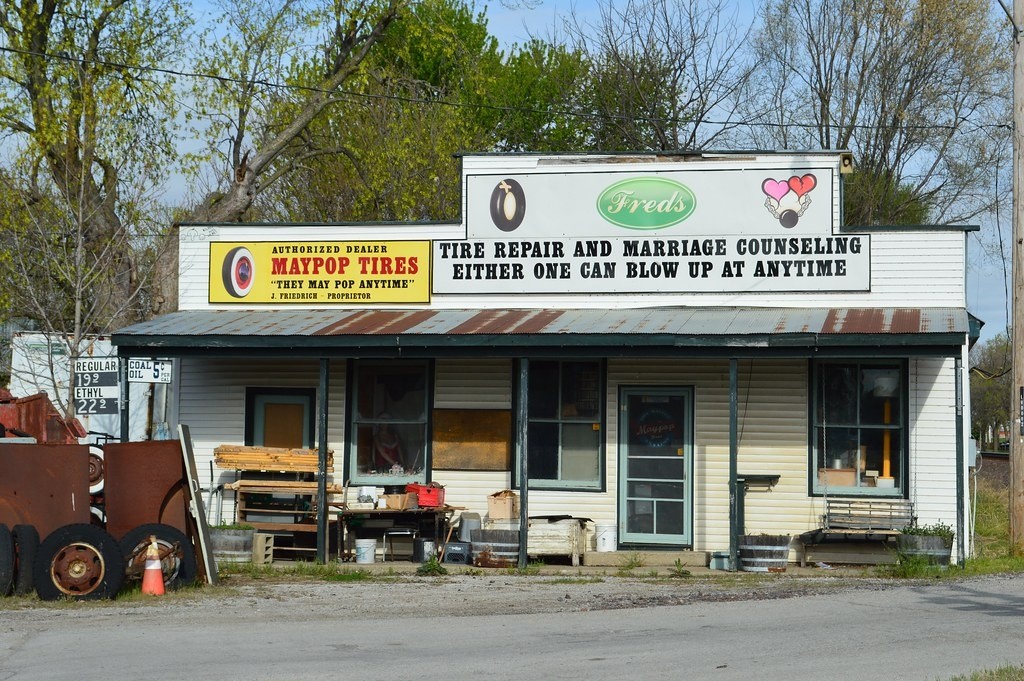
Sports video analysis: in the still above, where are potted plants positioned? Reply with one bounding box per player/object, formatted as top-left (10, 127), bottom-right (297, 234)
top-left (208, 517), bottom-right (258, 562)
top-left (896, 520), bottom-right (956, 570)
top-left (739, 532), bottom-right (793, 572)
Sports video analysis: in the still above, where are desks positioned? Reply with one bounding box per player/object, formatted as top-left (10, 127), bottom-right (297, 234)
top-left (819, 468), bottom-right (858, 487)
top-left (342, 510), bottom-right (447, 562)
top-left (529, 519), bottom-right (588, 564)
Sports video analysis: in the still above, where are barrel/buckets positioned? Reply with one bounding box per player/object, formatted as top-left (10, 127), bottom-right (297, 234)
top-left (355, 539), bottom-right (377, 563)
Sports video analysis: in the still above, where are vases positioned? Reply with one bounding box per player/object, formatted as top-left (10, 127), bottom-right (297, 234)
top-left (471, 528), bottom-right (520, 565)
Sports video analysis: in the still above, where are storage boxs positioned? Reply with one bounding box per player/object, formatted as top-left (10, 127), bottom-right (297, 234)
top-left (377, 494), bottom-right (406, 510)
top-left (348, 503), bottom-right (378, 510)
top-left (418, 487), bottom-right (446, 507)
top-left (443, 542), bottom-right (472, 563)
top-left (486, 496), bottom-right (521, 519)
top-left (865, 470), bottom-right (879, 477)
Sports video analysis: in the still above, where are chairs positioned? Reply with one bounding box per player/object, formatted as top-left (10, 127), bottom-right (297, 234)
top-left (382, 516), bottom-right (421, 561)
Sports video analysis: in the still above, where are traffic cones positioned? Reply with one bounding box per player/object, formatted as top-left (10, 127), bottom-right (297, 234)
top-left (141, 536), bottom-right (166, 596)
